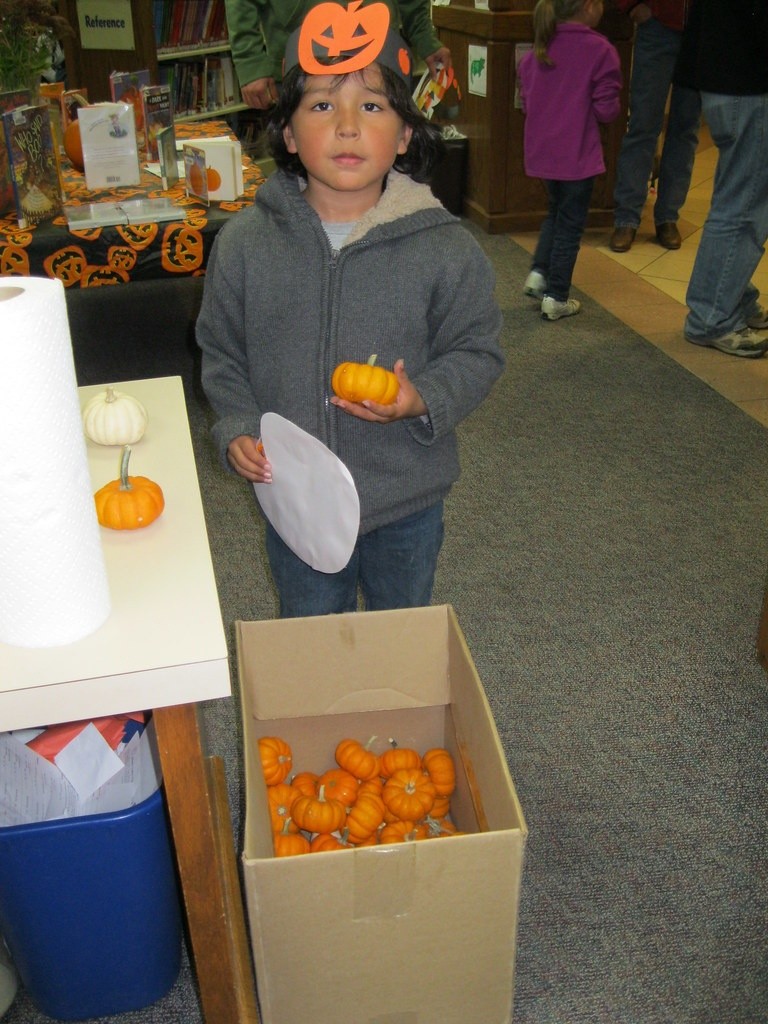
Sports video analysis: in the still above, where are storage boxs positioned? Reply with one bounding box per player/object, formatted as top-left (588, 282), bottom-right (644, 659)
top-left (234, 601), bottom-right (527, 1024)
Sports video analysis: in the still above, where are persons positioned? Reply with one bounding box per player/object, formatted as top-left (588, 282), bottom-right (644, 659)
top-left (225, 0), bottom-right (453, 108)
top-left (674, 0), bottom-right (768, 359)
top-left (194, 0), bottom-right (503, 619)
top-left (609, 1), bottom-right (701, 251)
top-left (516, 0), bottom-right (621, 320)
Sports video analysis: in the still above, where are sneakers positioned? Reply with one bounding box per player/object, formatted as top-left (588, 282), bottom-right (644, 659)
top-left (542, 295), bottom-right (580, 320)
top-left (684, 326), bottom-right (768, 357)
top-left (521, 269), bottom-right (547, 299)
top-left (745, 302), bottom-right (768, 328)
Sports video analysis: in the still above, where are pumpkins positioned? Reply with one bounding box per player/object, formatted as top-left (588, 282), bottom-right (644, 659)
top-left (119, 86), bottom-right (145, 131)
top-left (64, 93), bottom-right (90, 168)
top-left (96, 445), bottom-right (165, 530)
top-left (332, 353), bottom-right (399, 406)
top-left (190, 159), bottom-right (204, 195)
top-left (83, 386), bottom-right (147, 446)
top-left (205, 164), bottom-right (220, 192)
top-left (259, 732), bottom-right (469, 857)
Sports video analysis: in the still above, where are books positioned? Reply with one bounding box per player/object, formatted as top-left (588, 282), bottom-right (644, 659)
top-left (0, 69), bottom-right (244, 230)
top-left (151, 0), bottom-right (266, 159)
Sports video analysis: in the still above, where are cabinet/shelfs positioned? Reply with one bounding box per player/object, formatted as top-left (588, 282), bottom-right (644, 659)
top-left (67, 0), bottom-right (280, 179)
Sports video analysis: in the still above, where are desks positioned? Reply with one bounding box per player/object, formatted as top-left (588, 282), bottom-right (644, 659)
top-left (0, 375), bottom-right (261, 1024)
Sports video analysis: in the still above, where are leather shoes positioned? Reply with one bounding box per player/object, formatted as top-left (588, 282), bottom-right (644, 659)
top-left (656, 221), bottom-right (683, 249)
top-left (611, 223), bottom-right (636, 251)
